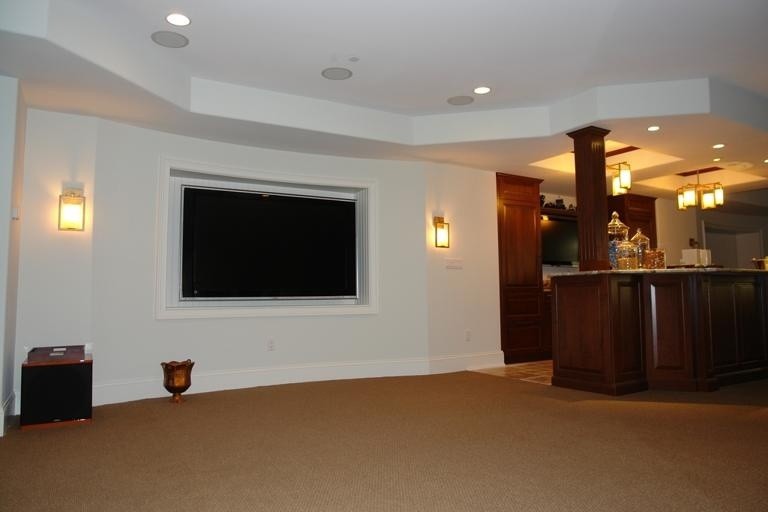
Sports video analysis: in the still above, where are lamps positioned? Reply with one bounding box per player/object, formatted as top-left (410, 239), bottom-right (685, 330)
top-left (57, 187), bottom-right (87, 231)
top-left (605, 161), bottom-right (632, 197)
top-left (676, 168), bottom-right (725, 211)
top-left (432, 215), bottom-right (450, 249)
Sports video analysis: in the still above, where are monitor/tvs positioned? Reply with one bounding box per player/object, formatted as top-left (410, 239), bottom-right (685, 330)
top-left (180, 185), bottom-right (359, 301)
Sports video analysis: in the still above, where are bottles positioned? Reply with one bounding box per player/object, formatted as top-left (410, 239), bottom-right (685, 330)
top-left (607, 211), bottom-right (650, 270)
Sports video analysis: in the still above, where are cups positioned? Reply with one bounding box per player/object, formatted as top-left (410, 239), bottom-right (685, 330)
top-left (642, 248), bottom-right (667, 270)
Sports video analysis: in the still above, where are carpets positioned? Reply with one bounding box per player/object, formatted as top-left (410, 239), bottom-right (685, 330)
top-left (12, 369), bottom-right (767, 512)
